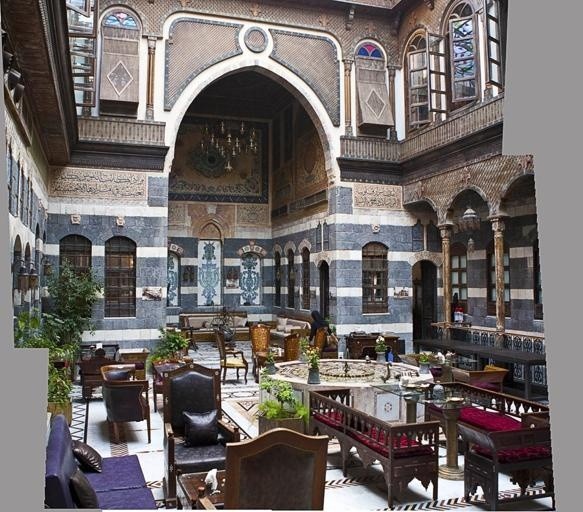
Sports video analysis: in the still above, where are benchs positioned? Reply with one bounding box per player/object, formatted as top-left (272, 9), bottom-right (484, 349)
top-left (422, 381), bottom-right (549, 449)
top-left (303, 384), bottom-right (442, 508)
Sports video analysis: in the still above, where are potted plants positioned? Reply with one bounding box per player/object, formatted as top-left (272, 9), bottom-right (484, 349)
top-left (16, 308), bottom-right (76, 428)
top-left (254, 364), bottom-right (309, 435)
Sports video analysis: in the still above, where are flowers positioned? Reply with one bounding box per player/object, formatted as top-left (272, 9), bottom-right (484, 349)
top-left (302, 348), bottom-right (319, 367)
top-left (374, 337), bottom-right (387, 354)
top-left (418, 348), bottom-right (429, 362)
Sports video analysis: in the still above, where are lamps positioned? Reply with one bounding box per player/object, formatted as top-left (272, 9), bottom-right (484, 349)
top-left (193, 74), bottom-right (263, 177)
top-left (9, 256), bottom-right (29, 296)
top-left (25, 260), bottom-right (39, 291)
top-left (40, 254), bottom-right (52, 276)
top-left (465, 236), bottom-right (478, 254)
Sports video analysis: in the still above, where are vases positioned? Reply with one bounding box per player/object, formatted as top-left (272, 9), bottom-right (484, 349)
top-left (417, 362), bottom-right (430, 374)
top-left (307, 366), bottom-right (321, 385)
top-left (376, 352), bottom-right (387, 364)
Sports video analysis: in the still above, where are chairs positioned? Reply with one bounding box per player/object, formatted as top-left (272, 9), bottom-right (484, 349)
top-left (248, 324), bottom-right (276, 376)
top-left (77, 337), bottom-right (191, 450)
top-left (194, 425), bottom-right (330, 509)
top-left (213, 329), bottom-right (249, 384)
top-left (304, 327), bottom-right (328, 358)
top-left (450, 362), bottom-right (508, 394)
top-left (160, 361), bottom-right (241, 508)
top-left (272, 332), bottom-right (302, 365)
top-left (462, 425), bottom-right (554, 510)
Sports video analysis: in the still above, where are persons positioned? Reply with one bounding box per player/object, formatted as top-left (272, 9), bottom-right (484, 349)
top-left (308, 310), bottom-right (331, 347)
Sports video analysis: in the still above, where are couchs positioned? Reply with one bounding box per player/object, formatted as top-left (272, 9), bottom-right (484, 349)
top-left (249, 314), bottom-right (314, 350)
top-left (40, 413), bottom-right (156, 508)
top-left (187, 316), bottom-right (250, 342)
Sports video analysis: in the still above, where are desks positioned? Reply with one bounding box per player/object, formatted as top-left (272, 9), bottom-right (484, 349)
top-left (344, 333), bottom-right (399, 361)
top-left (411, 338), bottom-right (546, 402)
top-left (372, 380), bottom-right (506, 482)
top-left (176, 466), bottom-right (226, 507)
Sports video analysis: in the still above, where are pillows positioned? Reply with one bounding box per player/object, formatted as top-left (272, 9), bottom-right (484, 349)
top-left (69, 468), bottom-right (100, 508)
top-left (179, 408), bottom-right (220, 447)
top-left (68, 435), bottom-right (104, 473)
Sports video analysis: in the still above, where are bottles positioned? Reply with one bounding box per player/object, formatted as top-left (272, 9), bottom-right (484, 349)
top-left (432, 380), bottom-right (444, 407)
